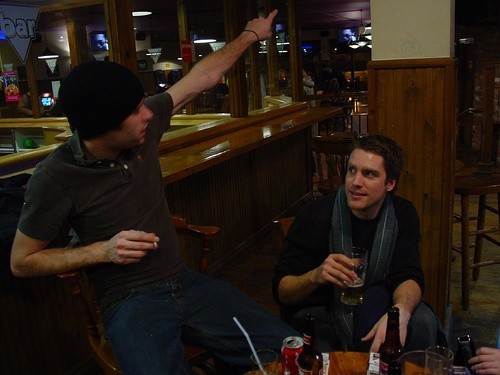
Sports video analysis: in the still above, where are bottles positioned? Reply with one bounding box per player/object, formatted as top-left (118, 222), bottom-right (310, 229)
top-left (296, 313), bottom-right (324, 375)
top-left (379, 306), bottom-right (404, 375)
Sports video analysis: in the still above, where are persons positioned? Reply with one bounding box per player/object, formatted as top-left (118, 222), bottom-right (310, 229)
top-left (267, 135), bottom-right (441, 355)
top-left (17, 90), bottom-right (43, 149)
top-left (9, 8), bottom-right (302, 375)
top-left (303, 69), bottom-right (318, 138)
top-left (320, 63), bottom-right (347, 92)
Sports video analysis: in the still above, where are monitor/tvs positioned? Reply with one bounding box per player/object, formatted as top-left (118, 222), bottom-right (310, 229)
top-left (338, 26), bottom-right (359, 43)
top-left (90, 32), bottom-right (109, 52)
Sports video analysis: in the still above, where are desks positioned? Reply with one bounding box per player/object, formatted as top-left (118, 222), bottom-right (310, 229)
top-left (246, 351), bottom-right (431, 375)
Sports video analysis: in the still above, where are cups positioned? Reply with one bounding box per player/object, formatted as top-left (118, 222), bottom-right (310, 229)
top-left (399, 345), bottom-right (470, 375)
top-left (341, 247), bottom-right (369, 305)
top-left (250, 348), bottom-right (278, 375)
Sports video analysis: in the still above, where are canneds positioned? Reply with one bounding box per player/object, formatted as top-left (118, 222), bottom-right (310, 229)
top-left (282, 336), bottom-right (303, 375)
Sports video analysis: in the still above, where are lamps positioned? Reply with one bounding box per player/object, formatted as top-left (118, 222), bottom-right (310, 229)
top-left (131, 9), bottom-right (153, 17)
top-left (192, 24), bottom-right (217, 44)
top-left (348, 44), bottom-right (360, 50)
top-left (365, 22), bottom-right (372, 30)
top-left (36, 31), bottom-right (60, 60)
top-left (354, 34), bottom-right (369, 47)
top-left (364, 34), bottom-right (371, 41)
top-left (366, 44), bottom-right (373, 49)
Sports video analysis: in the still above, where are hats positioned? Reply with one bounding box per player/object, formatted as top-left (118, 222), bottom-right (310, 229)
top-left (60, 61), bottom-right (145, 139)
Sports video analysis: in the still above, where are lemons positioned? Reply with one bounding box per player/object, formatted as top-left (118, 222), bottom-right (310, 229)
top-left (22, 137), bottom-right (34, 148)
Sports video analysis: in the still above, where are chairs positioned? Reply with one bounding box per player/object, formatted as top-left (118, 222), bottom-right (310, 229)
top-left (311, 132), bottom-right (357, 195)
top-left (52, 213), bottom-right (220, 375)
top-left (454, 106), bottom-right (500, 311)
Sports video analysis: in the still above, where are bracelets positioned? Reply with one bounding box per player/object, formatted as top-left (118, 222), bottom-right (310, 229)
top-left (244, 29), bottom-right (259, 40)
